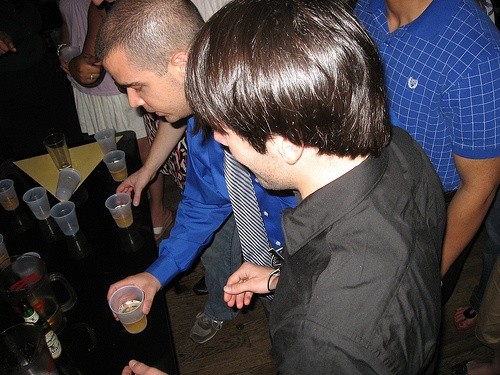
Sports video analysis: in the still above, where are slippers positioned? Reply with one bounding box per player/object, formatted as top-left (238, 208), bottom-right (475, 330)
top-left (446, 359), bottom-right (475, 375)
top-left (452, 305), bottom-right (477, 331)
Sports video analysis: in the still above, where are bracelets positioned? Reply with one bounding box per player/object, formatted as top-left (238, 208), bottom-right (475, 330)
top-left (57, 44), bottom-right (68, 56)
top-left (267, 269), bottom-right (280, 292)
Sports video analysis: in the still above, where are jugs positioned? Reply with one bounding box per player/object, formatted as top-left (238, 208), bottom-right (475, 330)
top-left (0, 322), bottom-right (60, 375)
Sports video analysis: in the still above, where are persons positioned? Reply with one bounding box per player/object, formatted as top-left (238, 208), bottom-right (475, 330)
top-left (452, 186), bottom-right (500, 375)
top-left (0, 0), bottom-right (208, 294)
top-left (96, 0), bottom-right (298, 345)
top-left (122, 0), bottom-right (447, 375)
top-left (354, 0), bottom-right (500, 279)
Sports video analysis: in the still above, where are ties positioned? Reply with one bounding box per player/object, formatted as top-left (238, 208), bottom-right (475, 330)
top-left (224, 147), bottom-right (276, 302)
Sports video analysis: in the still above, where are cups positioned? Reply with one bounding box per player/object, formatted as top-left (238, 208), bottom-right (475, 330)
top-left (43, 132), bottom-right (73, 171)
top-left (49, 201), bottom-right (80, 235)
top-left (0, 234), bottom-right (11, 270)
top-left (0, 254), bottom-right (78, 337)
top-left (94, 128), bottom-right (117, 154)
top-left (60, 45), bottom-right (81, 65)
top-left (105, 192), bottom-right (134, 228)
top-left (108, 286), bottom-right (148, 334)
top-left (0, 179), bottom-right (18, 211)
top-left (55, 168), bottom-right (82, 199)
top-left (103, 150), bottom-right (129, 182)
top-left (22, 251), bottom-right (40, 259)
top-left (22, 187), bottom-right (50, 220)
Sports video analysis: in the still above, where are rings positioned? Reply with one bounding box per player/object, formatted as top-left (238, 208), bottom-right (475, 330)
top-left (90, 74), bottom-right (93, 80)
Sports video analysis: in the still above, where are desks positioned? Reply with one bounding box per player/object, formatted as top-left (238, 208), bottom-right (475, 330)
top-left (0, 130), bottom-right (179, 375)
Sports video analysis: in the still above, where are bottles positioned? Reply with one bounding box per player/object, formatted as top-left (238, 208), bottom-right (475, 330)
top-left (15, 297), bottom-right (63, 361)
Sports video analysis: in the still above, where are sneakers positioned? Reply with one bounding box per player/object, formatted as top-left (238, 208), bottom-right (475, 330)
top-left (193, 276), bottom-right (209, 293)
top-left (190, 310), bottom-right (223, 344)
